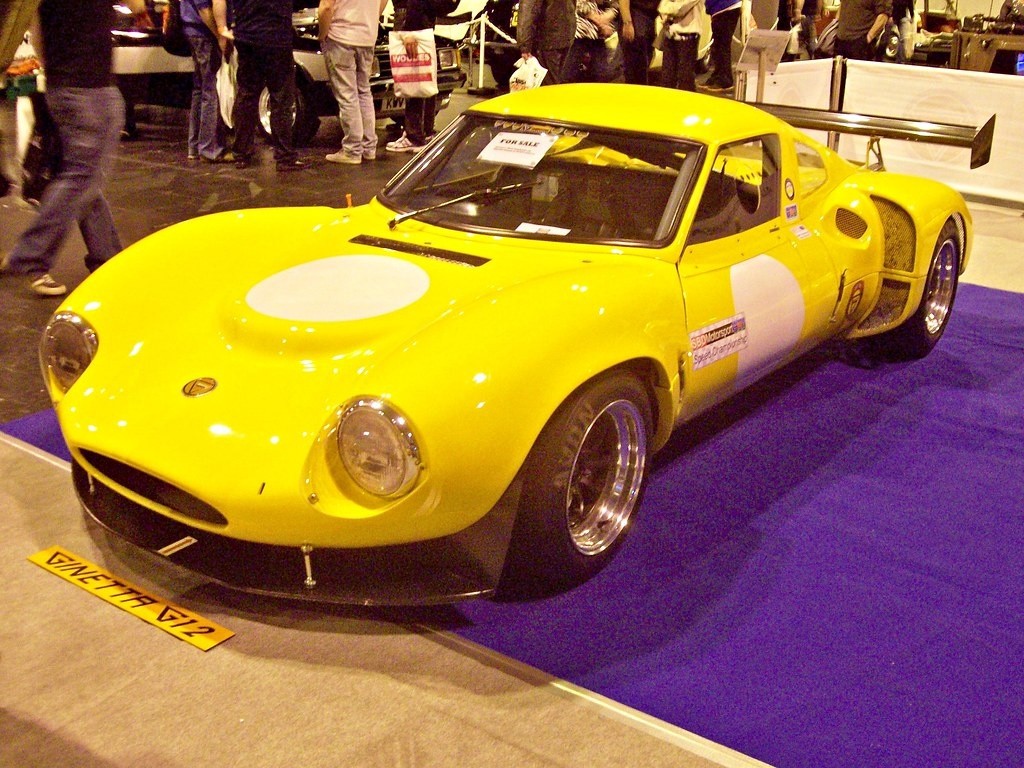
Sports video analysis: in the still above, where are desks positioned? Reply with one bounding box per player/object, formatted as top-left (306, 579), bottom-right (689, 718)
top-left (949, 31), bottom-right (1024, 73)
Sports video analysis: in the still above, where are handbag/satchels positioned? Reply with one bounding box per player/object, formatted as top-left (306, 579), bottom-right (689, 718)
top-left (388, 28), bottom-right (439, 99)
top-left (651, 22), bottom-right (667, 49)
top-left (508, 56), bottom-right (548, 92)
top-left (162, 0), bottom-right (194, 57)
top-left (214, 40), bottom-right (239, 128)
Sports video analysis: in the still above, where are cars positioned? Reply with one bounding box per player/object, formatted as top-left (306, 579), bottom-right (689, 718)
top-left (36, 82), bottom-right (999, 612)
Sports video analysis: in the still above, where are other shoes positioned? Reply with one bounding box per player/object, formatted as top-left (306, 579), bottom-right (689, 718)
top-left (698, 77), bottom-right (734, 91)
top-left (235, 156), bottom-right (261, 169)
top-left (276, 156), bottom-right (318, 169)
top-left (186, 146), bottom-right (235, 162)
top-left (1, 252), bottom-right (67, 296)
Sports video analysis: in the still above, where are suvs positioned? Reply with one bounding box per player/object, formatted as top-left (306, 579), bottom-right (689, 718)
top-left (112, 0), bottom-right (473, 150)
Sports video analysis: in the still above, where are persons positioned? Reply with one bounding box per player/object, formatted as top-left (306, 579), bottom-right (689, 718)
top-left (986, 0), bottom-right (1024, 37)
top-left (776, 1), bottom-right (917, 63)
top-left (656, 1), bottom-right (702, 92)
top-left (318, 0), bottom-right (387, 164)
top-left (0, 0), bottom-right (154, 296)
top-left (697, 0), bottom-right (743, 93)
top-left (386, 1), bottom-right (458, 153)
top-left (179, 0), bottom-right (236, 161)
top-left (517, 0), bottom-right (577, 87)
top-left (232, 0), bottom-right (313, 170)
top-left (570, 0), bottom-right (621, 83)
top-left (615, 0), bottom-right (660, 86)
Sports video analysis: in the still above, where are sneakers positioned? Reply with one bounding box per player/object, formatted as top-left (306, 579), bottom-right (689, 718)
top-left (386, 131), bottom-right (427, 154)
top-left (364, 153), bottom-right (376, 160)
top-left (425, 133), bottom-right (435, 140)
top-left (325, 150), bottom-right (362, 164)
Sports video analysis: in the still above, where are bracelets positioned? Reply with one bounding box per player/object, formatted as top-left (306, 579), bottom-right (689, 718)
top-left (622, 19), bottom-right (633, 25)
